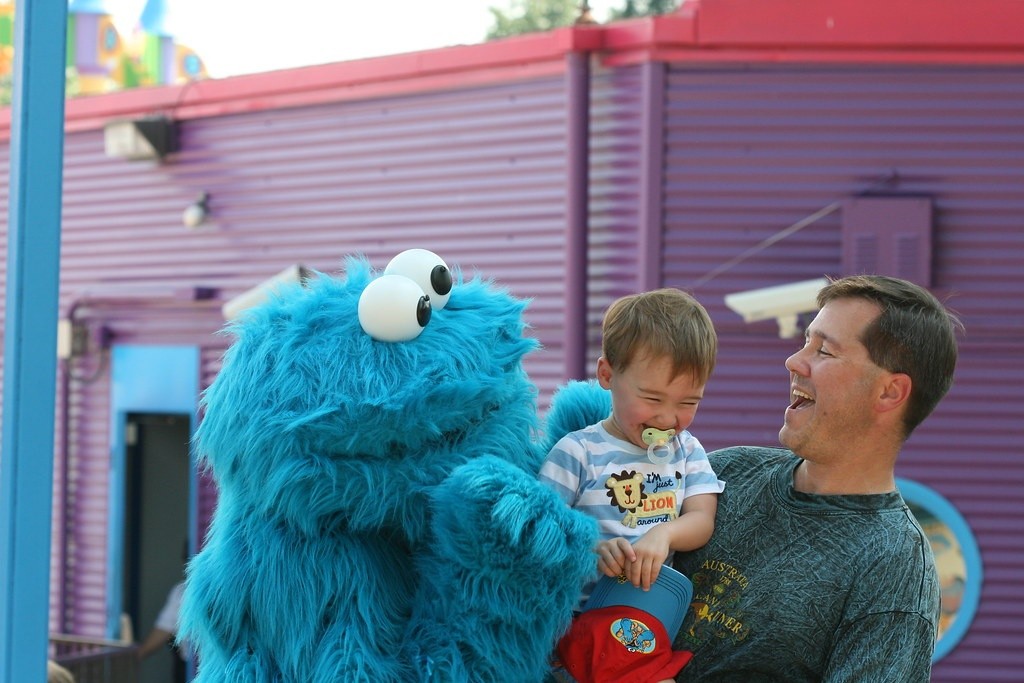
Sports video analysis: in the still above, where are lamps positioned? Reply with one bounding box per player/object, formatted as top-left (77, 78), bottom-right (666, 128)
top-left (182, 188), bottom-right (215, 231)
top-left (94, 114), bottom-right (183, 165)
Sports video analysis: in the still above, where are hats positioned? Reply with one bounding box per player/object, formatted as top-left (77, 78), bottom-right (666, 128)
top-left (557, 563), bottom-right (693, 683)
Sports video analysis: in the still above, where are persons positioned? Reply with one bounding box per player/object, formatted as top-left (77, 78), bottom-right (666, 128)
top-left (674, 275), bottom-right (965, 683)
top-left (535, 288), bottom-right (727, 683)
top-left (133, 542), bottom-right (190, 683)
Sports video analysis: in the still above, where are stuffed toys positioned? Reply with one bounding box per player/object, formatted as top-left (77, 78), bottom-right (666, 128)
top-left (173, 247), bottom-right (613, 683)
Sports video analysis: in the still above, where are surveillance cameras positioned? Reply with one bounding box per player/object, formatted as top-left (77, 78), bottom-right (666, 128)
top-left (723, 278), bottom-right (829, 323)
